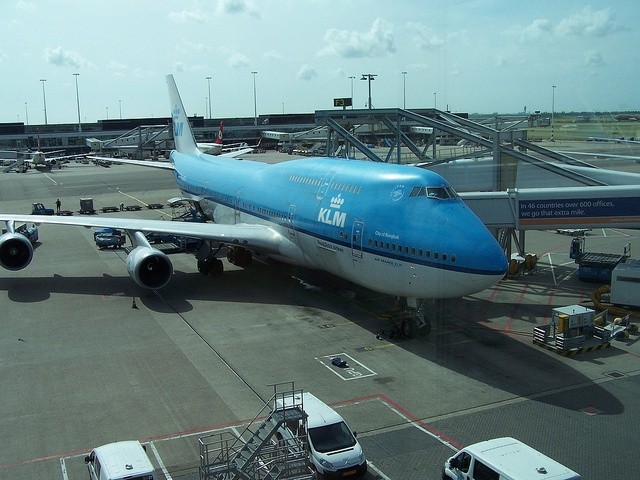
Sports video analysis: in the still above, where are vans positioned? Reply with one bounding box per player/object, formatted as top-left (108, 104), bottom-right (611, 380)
top-left (443, 436), bottom-right (581, 480)
top-left (273, 390), bottom-right (367, 479)
top-left (83, 438), bottom-right (156, 480)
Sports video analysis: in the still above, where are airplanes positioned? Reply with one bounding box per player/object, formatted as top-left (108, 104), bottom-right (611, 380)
top-left (1, 72), bottom-right (640, 265)
top-left (161, 119), bottom-right (259, 157)
top-left (613, 113), bottom-right (640, 122)
top-left (1, 133), bottom-right (95, 169)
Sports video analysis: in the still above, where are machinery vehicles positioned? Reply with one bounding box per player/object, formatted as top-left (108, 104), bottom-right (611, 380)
top-left (93, 227), bottom-right (126, 251)
top-left (31, 202), bottom-right (55, 215)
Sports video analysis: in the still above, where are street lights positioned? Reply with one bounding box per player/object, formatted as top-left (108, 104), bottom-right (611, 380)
top-left (251, 72), bottom-right (258, 126)
top-left (40, 79), bottom-right (47, 124)
top-left (206, 76), bottom-right (212, 119)
top-left (551, 85), bottom-right (557, 142)
top-left (347, 77), bottom-right (356, 110)
top-left (402, 73), bottom-right (407, 110)
top-left (73, 73), bottom-right (82, 132)
top-left (24, 102), bottom-right (29, 125)
top-left (360, 74), bottom-right (378, 110)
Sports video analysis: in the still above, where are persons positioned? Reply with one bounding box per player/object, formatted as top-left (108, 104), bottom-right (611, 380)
top-left (119, 202), bottom-right (124, 211)
top-left (55, 198), bottom-right (61, 212)
